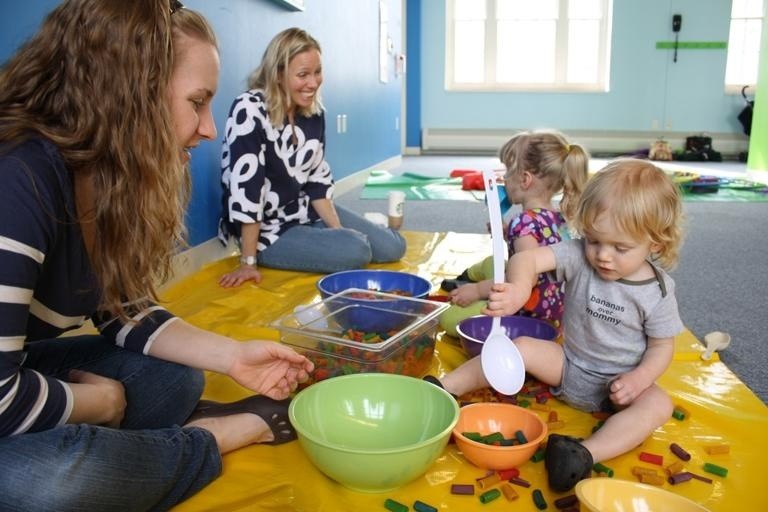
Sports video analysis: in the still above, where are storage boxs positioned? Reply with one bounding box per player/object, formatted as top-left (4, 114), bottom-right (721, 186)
top-left (273, 285), bottom-right (454, 382)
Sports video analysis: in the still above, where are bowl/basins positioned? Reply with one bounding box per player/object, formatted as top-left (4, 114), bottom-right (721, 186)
top-left (315, 269), bottom-right (434, 334)
top-left (287, 373), bottom-right (460, 495)
top-left (440, 299), bottom-right (560, 358)
top-left (575, 477), bottom-right (713, 512)
top-left (452, 403), bottom-right (547, 471)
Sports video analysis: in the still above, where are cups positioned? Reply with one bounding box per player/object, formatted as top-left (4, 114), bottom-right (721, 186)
top-left (388, 191), bottom-right (406, 230)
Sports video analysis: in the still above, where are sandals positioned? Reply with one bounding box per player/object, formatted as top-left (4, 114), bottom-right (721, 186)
top-left (180, 392), bottom-right (299, 446)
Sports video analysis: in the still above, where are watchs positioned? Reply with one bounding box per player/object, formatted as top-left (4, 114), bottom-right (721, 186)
top-left (238, 256), bottom-right (258, 266)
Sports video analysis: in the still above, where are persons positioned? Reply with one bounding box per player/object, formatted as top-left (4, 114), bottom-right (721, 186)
top-left (216, 26), bottom-right (407, 289)
top-left (442, 131), bottom-right (590, 339)
top-left (0, 0), bottom-right (315, 512)
top-left (419, 158), bottom-right (687, 492)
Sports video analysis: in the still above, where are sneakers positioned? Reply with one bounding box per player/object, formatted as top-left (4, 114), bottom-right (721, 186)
top-left (544, 433), bottom-right (594, 493)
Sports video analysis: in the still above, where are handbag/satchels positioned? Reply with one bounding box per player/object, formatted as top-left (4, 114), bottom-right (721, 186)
top-left (649, 139), bottom-right (673, 161)
